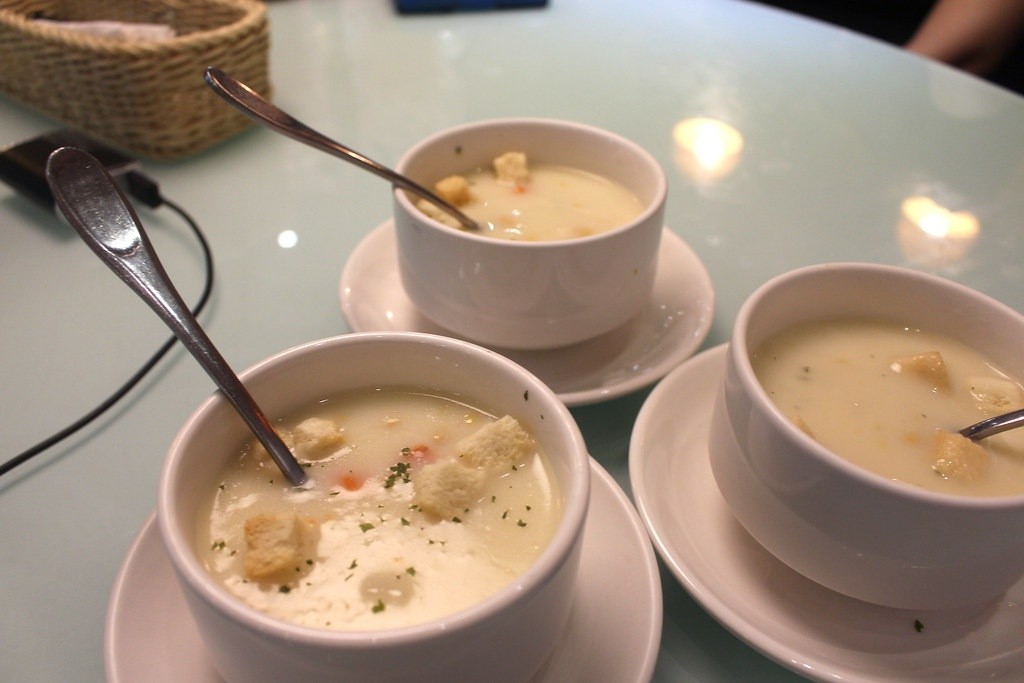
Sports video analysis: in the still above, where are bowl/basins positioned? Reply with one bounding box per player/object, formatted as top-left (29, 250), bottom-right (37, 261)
top-left (707, 260), bottom-right (1024, 612)
top-left (392, 118), bottom-right (668, 350)
top-left (157, 330), bottom-right (590, 683)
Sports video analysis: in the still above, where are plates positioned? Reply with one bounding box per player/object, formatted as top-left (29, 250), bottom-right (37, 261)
top-left (337, 216), bottom-right (716, 407)
top-left (627, 340), bottom-right (1023, 683)
top-left (104, 449), bottom-right (665, 682)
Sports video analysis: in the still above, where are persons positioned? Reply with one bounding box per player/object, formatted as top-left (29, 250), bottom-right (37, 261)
top-left (755, 0), bottom-right (1024, 78)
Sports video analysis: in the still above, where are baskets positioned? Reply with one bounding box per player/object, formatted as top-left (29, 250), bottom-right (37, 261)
top-left (0, 0), bottom-right (272, 161)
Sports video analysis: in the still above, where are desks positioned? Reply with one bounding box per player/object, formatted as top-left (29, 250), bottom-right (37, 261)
top-left (0, 2), bottom-right (1024, 683)
